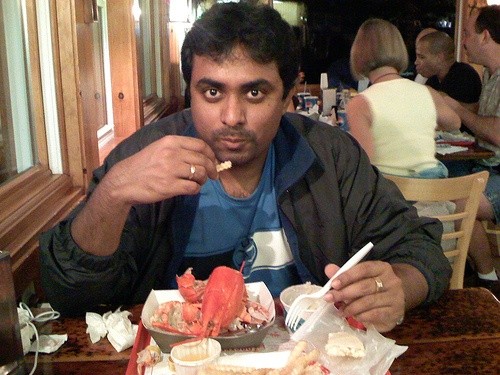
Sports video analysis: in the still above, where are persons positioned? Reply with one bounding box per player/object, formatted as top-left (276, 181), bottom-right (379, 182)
top-left (40, 0), bottom-right (500, 336)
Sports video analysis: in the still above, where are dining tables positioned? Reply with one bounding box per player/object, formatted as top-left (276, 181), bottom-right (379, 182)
top-left (25, 288), bottom-right (500, 375)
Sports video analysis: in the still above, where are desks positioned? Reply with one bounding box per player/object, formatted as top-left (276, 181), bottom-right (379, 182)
top-left (436, 142), bottom-right (496, 159)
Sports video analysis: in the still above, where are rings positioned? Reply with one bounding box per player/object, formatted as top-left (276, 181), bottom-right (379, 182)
top-left (187, 163), bottom-right (196, 180)
top-left (372, 275), bottom-right (384, 294)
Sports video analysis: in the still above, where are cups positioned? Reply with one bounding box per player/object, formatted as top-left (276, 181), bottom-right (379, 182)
top-left (280, 284), bottom-right (322, 334)
top-left (171, 337), bottom-right (222, 375)
top-left (303, 96), bottom-right (317, 112)
top-left (297, 92), bottom-right (310, 111)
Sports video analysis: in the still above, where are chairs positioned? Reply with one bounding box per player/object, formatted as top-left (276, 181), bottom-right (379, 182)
top-left (381, 171), bottom-right (489, 291)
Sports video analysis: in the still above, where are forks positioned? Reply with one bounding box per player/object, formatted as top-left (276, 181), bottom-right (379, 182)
top-left (285, 241), bottom-right (374, 330)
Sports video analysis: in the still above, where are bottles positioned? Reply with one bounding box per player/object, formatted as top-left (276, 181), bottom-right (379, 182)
top-left (337, 89), bottom-right (353, 132)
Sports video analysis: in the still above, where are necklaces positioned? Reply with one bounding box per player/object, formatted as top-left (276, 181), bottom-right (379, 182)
top-left (372, 72), bottom-right (399, 84)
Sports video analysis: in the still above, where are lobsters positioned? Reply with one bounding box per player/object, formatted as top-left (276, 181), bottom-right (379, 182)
top-left (151, 265), bottom-right (270, 350)
